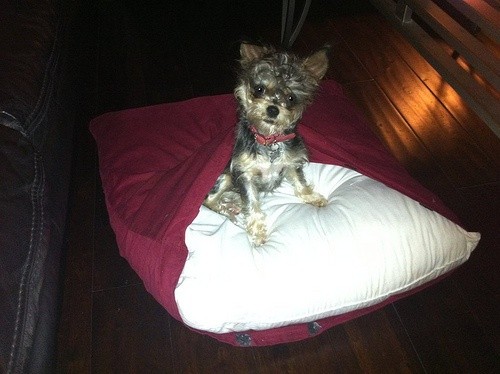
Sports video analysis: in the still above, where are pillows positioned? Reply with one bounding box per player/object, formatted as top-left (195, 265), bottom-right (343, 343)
top-left (87, 78), bottom-right (482, 346)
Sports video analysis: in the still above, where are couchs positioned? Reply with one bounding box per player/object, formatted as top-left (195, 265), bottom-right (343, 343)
top-left (2, 0), bottom-right (76, 365)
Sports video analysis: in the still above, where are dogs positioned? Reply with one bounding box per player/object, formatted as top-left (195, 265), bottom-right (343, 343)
top-left (203, 40), bottom-right (330, 247)
